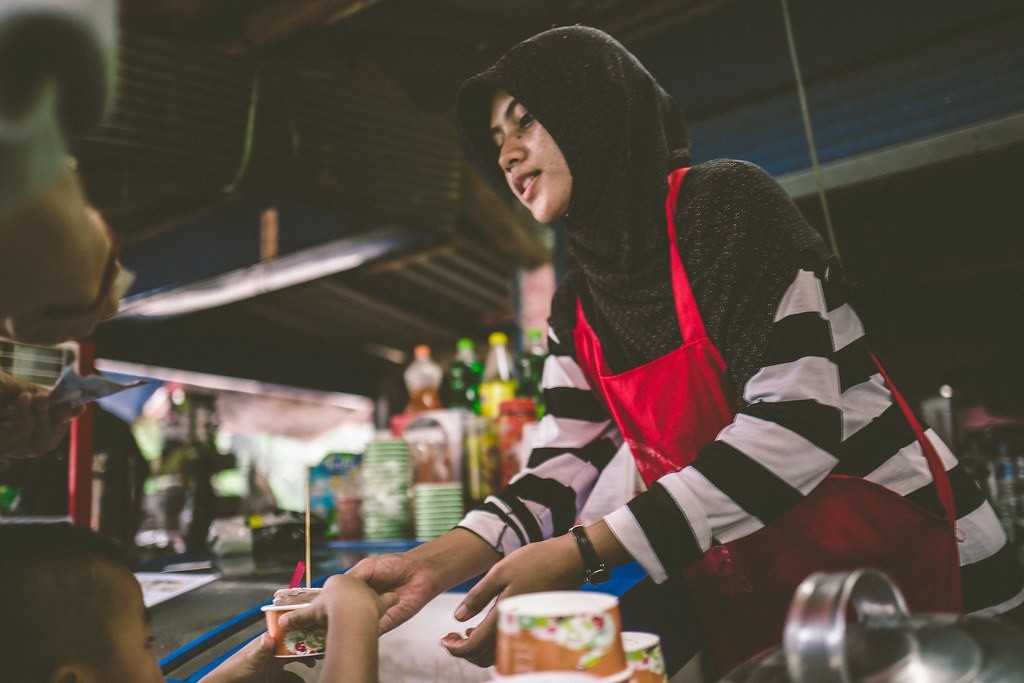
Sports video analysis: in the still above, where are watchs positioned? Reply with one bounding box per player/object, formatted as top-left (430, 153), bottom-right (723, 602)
top-left (567, 520), bottom-right (612, 586)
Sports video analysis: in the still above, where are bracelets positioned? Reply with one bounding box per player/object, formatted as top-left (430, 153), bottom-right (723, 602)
top-left (37, 222), bottom-right (120, 320)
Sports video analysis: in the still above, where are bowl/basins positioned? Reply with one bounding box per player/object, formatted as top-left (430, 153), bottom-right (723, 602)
top-left (264, 600), bottom-right (328, 662)
top-left (618, 627), bottom-right (669, 683)
top-left (493, 586), bottom-right (636, 683)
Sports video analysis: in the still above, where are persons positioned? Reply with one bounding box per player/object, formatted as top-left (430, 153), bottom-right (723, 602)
top-left (1, 0), bottom-right (126, 457)
top-left (328, 24), bottom-right (1023, 682)
top-left (1, 519), bottom-right (400, 682)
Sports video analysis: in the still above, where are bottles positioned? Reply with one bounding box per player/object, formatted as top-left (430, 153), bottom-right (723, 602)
top-left (332, 324), bottom-right (549, 544)
